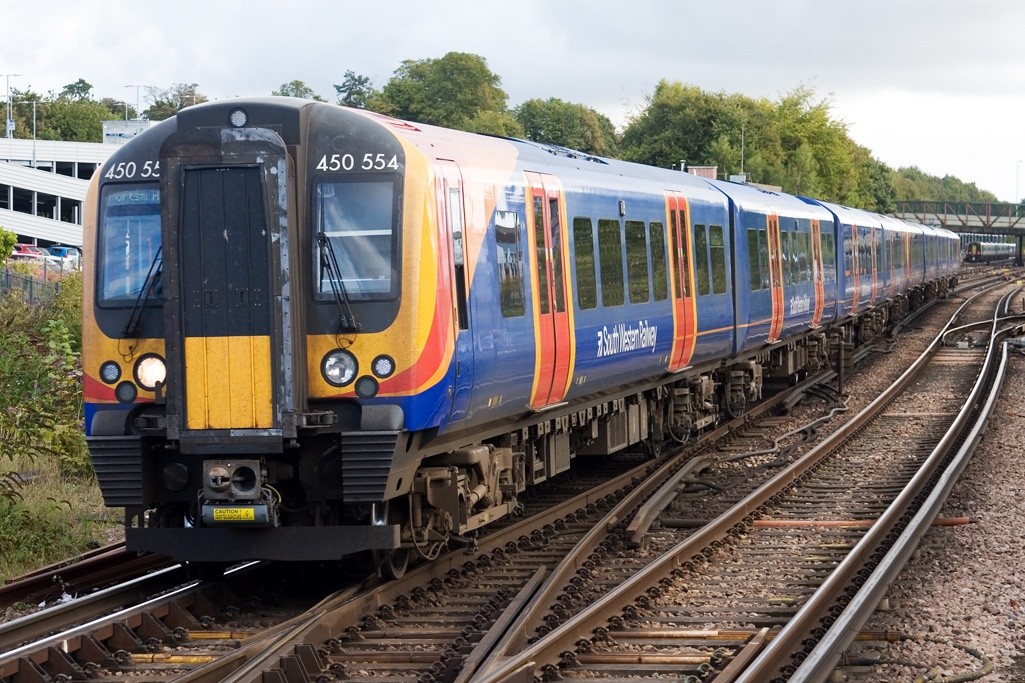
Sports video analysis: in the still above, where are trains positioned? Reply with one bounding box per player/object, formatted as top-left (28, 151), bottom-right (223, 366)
top-left (79, 94), bottom-right (960, 581)
top-left (965, 241), bottom-right (1016, 263)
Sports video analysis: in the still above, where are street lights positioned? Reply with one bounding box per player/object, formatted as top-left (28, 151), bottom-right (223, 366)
top-left (740, 122), bottom-right (747, 182)
top-left (124, 83), bottom-right (153, 136)
top-left (0, 73), bottom-right (23, 138)
top-left (0, 94), bottom-right (27, 138)
top-left (112, 102), bottom-right (137, 121)
top-left (1016, 159), bottom-right (1024, 216)
top-left (181, 95), bottom-right (208, 105)
top-left (16, 100), bottom-right (51, 246)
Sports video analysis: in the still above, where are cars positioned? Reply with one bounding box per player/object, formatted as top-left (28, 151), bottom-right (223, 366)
top-left (3, 243), bottom-right (83, 296)
